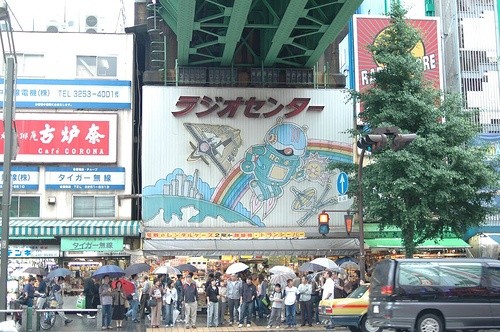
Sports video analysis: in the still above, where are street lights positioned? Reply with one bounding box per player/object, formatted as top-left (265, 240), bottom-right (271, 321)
top-left (0, 2), bottom-right (20, 323)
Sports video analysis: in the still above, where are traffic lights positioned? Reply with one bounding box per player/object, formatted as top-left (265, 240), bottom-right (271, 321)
top-left (357, 133), bottom-right (387, 154)
top-left (318, 214), bottom-right (329, 234)
top-left (388, 134), bottom-right (416, 152)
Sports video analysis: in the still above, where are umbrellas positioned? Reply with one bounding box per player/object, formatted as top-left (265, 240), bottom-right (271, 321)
top-left (153, 266), bottom-right (181, 275)
top-left (178, 264), bottom-right (197, 272)
top-left (270, 265), bottom-right (296, 285)
top-left (24, 267), bottom-right (49, 276)
top-left (47, 267), bottom-right (74, 279)
top-left (92, 265), bottom-right (125, 278)
top-left (125, 263), bottom-right (150, 274)
top-left (300, 256), bottom-right (358, 273)
top-left (226, 263), bottom-right (249, 274)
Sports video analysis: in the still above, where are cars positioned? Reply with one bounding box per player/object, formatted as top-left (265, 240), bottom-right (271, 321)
top-left (318, 284), bottom-right (383, 332)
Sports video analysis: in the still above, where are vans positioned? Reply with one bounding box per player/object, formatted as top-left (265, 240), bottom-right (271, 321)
top-left (368, 257), bottom-right (500, 332)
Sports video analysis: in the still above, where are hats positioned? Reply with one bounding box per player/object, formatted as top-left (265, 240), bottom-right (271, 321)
top-left (187, 274), bottom-right (192, 278)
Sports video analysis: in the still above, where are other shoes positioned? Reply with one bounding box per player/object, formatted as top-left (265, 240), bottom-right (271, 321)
top-left (265, 320), bottom-right (331, 329)
top-left (146, 315), bottom-right (251, 329)
top-left (103, 326), bottom-right (107, 329)
top-left (123, 314), bottom-right (128, 321)
top-left (77, 313), bottom-right (83, 319)
top-left (86, 314), bottom-right (95, 319)
top-left (116, 325), bottom-right (123, 328)
top-left (108, 326), bottom-right (113, 329)
top-left (133, 319), bottom-right (141, 324)
top-left (44, 319), bottom-right (52, 325)
top-left (64, 319), bottom-right (72, 325)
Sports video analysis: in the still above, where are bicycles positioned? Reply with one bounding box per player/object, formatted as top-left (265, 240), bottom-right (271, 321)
top-left (17, 293), bottom-right (56, 330)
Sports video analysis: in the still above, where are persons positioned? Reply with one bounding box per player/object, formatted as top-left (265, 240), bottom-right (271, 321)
top-left (82, 272), bottom-right (198, 330)
top-left (22, 274), bottom-right (73, 324)
top-left (204, 270), bottom-right (360, 328)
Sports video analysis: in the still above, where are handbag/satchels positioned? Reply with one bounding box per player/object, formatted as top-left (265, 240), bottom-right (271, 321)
top-left (260, 298), bottom-right (268, 306)
top-left (237, 305), bottom-right (243, 320)
top-left (17, 294), bottom-right (27, 304)
top-left (124, 298), bottom-right (130, 308)
top-left (146, 299), bottom-right (156, 306)
top-left (76, 294), bottom-right (85, 309)
top-left (35, 296), bottom-right (46, 310)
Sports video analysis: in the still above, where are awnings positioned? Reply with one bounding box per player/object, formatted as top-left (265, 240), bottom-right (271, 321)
top-left (9, 219), bottom-right (140, 236)
top-left (364, 238), bottom-right (473, 248)
top-left (142, 239), bottom-right (360, 256)
